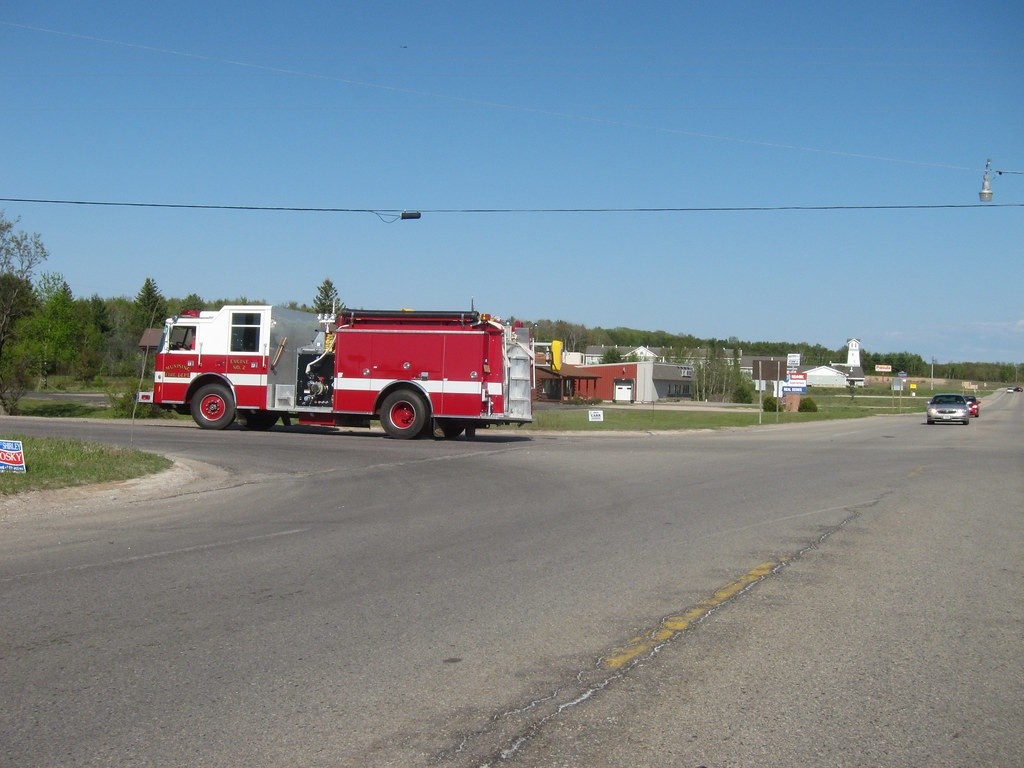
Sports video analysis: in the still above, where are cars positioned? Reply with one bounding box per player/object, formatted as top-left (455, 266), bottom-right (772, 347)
top-left (1013, 387), bottom-right (1023, 392)
top-left (1006, 387), bottom-right (1014, 393)
top-left (956, 395), bottom-right (981, 417)
top-left (926, 393), bottom-right (972, 425)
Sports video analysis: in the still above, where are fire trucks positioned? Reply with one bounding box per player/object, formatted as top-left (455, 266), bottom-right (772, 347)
top-left (138, 296), bottom-right (536, 440)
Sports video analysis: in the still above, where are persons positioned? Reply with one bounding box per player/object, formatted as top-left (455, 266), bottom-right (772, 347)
top-left (179, 329), bottom-right (195, 351)
